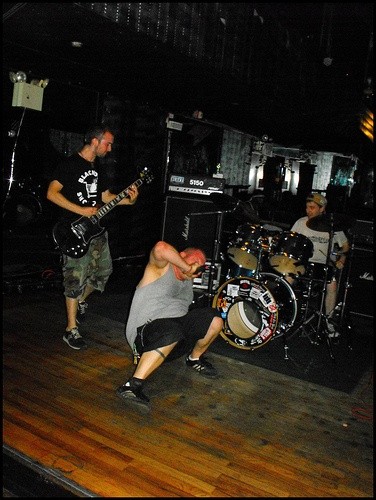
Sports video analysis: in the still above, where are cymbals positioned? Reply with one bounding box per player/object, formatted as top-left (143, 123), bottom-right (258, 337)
top-left (306, 215), bottom-right (355, 231)
top-left (209, 192), bottom-right (257, 221)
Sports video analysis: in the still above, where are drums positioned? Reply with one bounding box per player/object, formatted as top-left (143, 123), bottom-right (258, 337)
top-left (269, 231), bottom-right (314, 277)
top-left (212, 273), bottom-right (298, 351)
top-left (299, 261), bottom-right (333, 283)
top-left (226, 224), bottom-right (269, 272)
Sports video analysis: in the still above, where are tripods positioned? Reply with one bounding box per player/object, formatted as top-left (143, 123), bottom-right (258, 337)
top-left (279, 224), bottom-right (353, 362)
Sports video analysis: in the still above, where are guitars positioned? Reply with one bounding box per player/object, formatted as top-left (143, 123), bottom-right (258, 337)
top-left (52, 166), bottom-right (154, 258)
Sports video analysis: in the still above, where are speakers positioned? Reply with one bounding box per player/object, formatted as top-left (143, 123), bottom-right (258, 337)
top-left (163, 197), bottom-right (244, 261)
top-left (335, 247), bottom-right (376, 318)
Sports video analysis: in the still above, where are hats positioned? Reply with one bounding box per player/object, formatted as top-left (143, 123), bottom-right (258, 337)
top-left (307, 193), bottom-right (327, 207)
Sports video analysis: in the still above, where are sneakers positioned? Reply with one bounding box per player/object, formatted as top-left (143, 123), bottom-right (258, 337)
top-left (63, 327), bottom-right (88, 351)
top-left (187, 358), bottom-right (218, 379)
top-left (75, 303), bottom-right (88, 325)
top-left (324, 314), bottom-right (340, 338)
top-left (116, 383), bottom-right (151, 414)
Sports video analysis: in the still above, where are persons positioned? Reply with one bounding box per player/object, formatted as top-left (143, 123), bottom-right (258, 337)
top-left (47, 125), bottom-right (137, 349)
top-left (119, 241), bottom-right (222, 402)
top-left (290, 194), bottom-right (349, 332)
